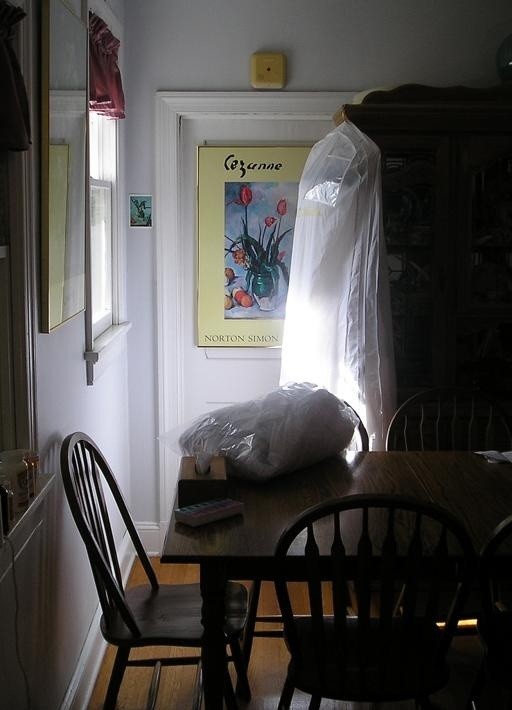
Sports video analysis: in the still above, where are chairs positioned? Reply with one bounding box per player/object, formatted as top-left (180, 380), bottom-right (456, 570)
top-left (231, 391), bottom-right (372, 695)
top-left (63, 435), bottom-right (254, 705)
top-left (386, 386), bottom-right (510, 452)
top-left (271, 491), bottom-right (480, 705)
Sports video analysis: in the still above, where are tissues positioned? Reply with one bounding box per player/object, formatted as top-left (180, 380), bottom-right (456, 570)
top-left (176, 447), bottom-right (228, 507)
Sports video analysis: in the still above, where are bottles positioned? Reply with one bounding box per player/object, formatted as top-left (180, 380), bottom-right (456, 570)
top-left (9, 453), bottom-right (28, 514)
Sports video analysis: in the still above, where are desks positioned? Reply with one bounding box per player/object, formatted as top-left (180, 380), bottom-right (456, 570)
top-left (157, 449), bottom-right (510, 568)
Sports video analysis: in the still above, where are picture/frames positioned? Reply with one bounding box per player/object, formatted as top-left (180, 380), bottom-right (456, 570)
top-left (129, 193), bottom-right (152, 228)
top-left (194, 145), bottom-right (315, 348)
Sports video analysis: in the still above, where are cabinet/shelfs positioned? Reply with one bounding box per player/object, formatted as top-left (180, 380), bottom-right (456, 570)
top-left (334, 85), bottom-right (511, 449)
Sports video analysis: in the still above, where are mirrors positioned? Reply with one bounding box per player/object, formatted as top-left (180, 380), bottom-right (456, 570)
top-left (41, 2), bottom-right (93, 330)
